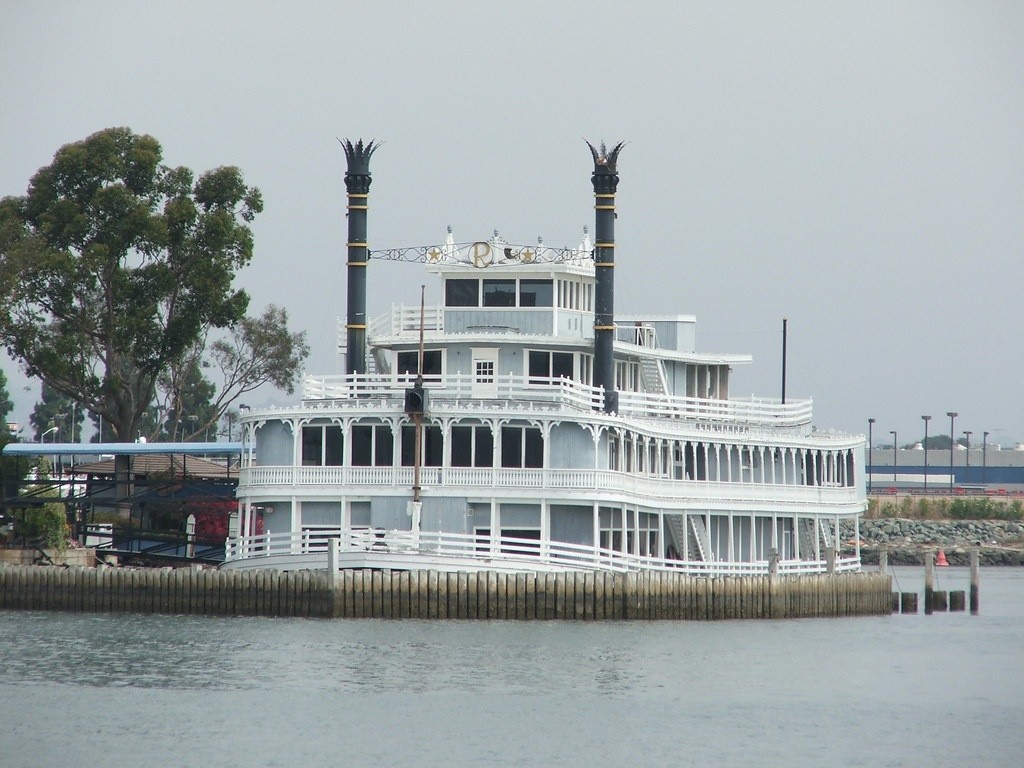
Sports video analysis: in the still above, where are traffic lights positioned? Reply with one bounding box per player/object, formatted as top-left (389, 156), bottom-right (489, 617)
top-left (404, 387), bottom-right (431, 415)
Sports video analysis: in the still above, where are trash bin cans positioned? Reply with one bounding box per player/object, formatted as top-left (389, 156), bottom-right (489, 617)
top-left (85, 522), bottom-right (114, 548)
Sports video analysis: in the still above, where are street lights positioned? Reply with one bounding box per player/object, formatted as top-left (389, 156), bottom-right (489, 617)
top-left (41, 427), bottom-right (59, 443)
top-left (889, 431), bottom-right (898, 480)
top-left (983, 431), bottom-right (989, 482)
top-left (946, 412), bottom-right (959, 492)
top-left (963, 431), bottom-right (973, 484)
top-left (867, 418), bottom-right (875, 489)
top-left (921, 415), bottom-right (932, 490)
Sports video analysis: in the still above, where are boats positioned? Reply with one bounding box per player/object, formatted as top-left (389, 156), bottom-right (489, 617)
top-left (219, 137), bottom-right (869, 576)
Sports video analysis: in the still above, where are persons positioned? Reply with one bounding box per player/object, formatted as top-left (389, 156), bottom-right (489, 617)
top-left (373, 527), bottom-right (389, 551)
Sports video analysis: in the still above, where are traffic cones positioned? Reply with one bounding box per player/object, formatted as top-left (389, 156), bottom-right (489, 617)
top-left (933, 546), bottom-right (953, 568)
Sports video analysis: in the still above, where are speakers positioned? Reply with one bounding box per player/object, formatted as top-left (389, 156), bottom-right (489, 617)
top-left (405, 388), bottom-right (429, 412)
